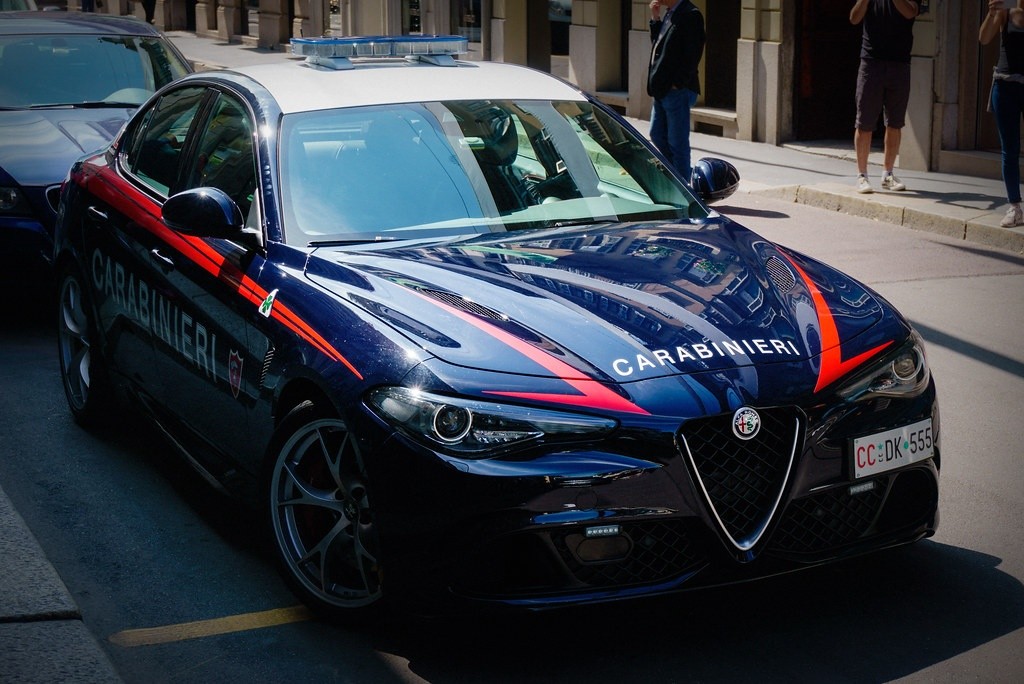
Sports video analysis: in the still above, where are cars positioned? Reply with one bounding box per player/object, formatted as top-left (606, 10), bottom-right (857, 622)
top-left (0, 12), bottom-right (194, 310)
top-left (63, 34), bottom-right (945, 630)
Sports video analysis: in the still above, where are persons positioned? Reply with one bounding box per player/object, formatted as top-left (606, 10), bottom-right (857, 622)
top-left (82, 0), bottom-right (95, 13)
top-left (140, 0), bottom-right (157, 25)
top-left (850, 0), bottom-right (919, 195)
top-left (978, 0), bottom-right (1024, 230)
top-left (414, 118), bottom-right (545, 226)
top-left (646, 0), bottom-right (705, 186)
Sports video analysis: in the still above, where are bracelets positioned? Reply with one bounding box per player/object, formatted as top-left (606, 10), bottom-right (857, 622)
top-left (988, 11), bottom-right (995, 18)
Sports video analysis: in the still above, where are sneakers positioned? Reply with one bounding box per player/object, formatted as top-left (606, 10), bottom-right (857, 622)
top-left (857, 174), bottom-right (874, 193)
top-left (1000, 206), bottom-right (1024, 227)
top-left (881, 175), bottom-right (906, 190)
